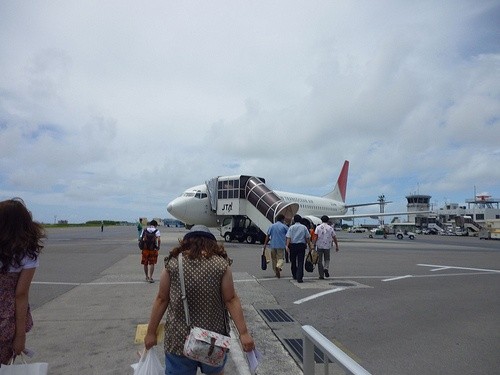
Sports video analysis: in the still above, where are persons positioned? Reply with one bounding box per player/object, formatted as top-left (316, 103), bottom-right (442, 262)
top-left (309, 224), bottom-right (317, 251)
top-left (101, 223), bottom-right (104, 232)
top-left (138, 220), bottom-right (161, 283)
top-left (311, 215), bottom-right (339, 279)
top-left (263, 215), bottom-right (289, 278)
top-left (0, 197), bottom-right (48, 368)
top-left (144, 225), bottom-right (255, 375)
top-left (285, 215), bottom-right (312, 283)
top-left (136, 218), bottom-right (142, 240)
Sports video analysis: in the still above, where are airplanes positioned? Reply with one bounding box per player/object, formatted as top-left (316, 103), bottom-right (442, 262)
top-left (166, 160), bottom-right (433, 240)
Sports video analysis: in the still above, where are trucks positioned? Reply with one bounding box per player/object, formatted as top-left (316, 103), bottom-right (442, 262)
top-left (216, 215), bottom-right (270, 244)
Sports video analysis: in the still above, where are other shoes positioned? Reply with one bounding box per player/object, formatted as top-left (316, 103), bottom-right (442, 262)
top-left (275, 267), bottom-right (280, 278)
top-left (144, 277), bottom-right (154, 283)
top-left (293, 277), bottom-right (303, 282)
top-left (324, 269), bottom-right (329, 277)
top-left (319, 277), bottom-right (324, 279)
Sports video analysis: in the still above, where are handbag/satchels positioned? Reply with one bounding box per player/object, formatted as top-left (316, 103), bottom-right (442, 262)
top-left (261, 252), bottom-right (267, 270)
top-left (305, 251), bottom-right (313, 273)
top-left (311, 249), bottom-right (319, 264)
top-left (182, 327), bottom-right (232, 368)
top-left (0, 350), bottom-right (48, 375)
top-left (133, 347), bottom-right (165, 375)
top-left (265, 248), bottom-right (271, 262)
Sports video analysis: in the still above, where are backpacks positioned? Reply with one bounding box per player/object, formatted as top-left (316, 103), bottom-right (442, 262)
top-left (138, 228), bottom-right (158, 251)
